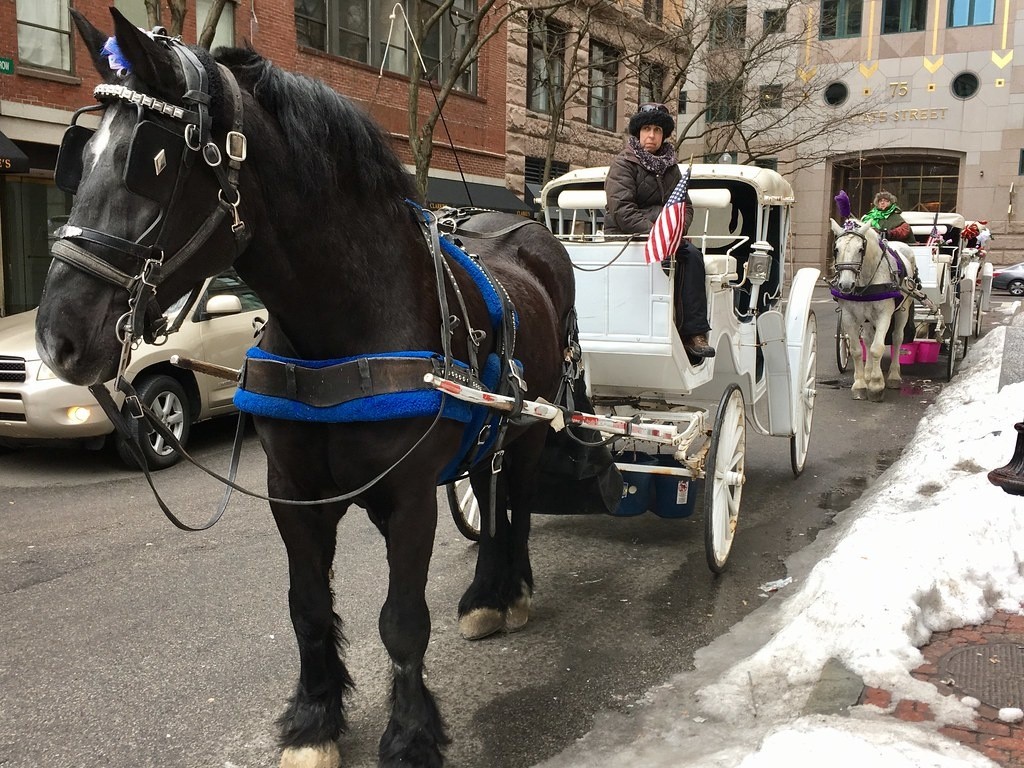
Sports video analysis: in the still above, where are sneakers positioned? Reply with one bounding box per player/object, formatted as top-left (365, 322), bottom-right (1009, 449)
top-left (680, 333), bottom-right (715, 357)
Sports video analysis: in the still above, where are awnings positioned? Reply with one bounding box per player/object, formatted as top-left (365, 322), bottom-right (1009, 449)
top-left (0, 130), bottom-right (30, 175)
top-left (410, 174), bottom-right (534, 220)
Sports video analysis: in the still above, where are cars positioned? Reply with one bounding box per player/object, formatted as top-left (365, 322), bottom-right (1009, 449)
top-left (992, 261), bottom-right (1024, 298)
top-left (0, 266), bottom-right (270, 472)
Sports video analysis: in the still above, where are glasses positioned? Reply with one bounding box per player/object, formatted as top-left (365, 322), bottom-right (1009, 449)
top-left (640, 105), bottom-right (669, 114)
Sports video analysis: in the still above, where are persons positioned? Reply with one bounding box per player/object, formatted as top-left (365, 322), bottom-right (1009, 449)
top-left (603, 103), bottom-right (715, 364)
top-left (864, 191), bottom-right (916, 243)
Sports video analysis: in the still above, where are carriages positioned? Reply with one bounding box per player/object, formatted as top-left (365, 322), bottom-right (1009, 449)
top-left (26, 4), bottom-right (829, 768)
top-left (821, 187), bottom-right (995, 404)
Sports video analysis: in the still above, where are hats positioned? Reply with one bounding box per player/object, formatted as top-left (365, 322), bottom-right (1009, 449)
top-left (874, 191), bottom-right (898, 207)
top-left (629, 103), bottom-right (674, 141)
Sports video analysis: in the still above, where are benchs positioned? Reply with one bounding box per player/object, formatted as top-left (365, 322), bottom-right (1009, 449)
top-left (558, 189), bottom-right (751, 284)
top-left (909, 225), bottom-right (959, 265)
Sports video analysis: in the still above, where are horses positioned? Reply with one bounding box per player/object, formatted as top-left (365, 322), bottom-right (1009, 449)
top-left (828, 217), bottom-right (916, 403)
top-left (32, 5), bottom-right (582, 768)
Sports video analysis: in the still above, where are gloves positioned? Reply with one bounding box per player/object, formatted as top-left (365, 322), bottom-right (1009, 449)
top-left (878, 229), bottom-right (890, 240)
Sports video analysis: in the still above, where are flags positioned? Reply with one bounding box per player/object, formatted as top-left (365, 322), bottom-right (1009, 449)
top-left (926, 226), bottom-right (938, 254)
top-left (645, 167), bottom-right (690, 265)
